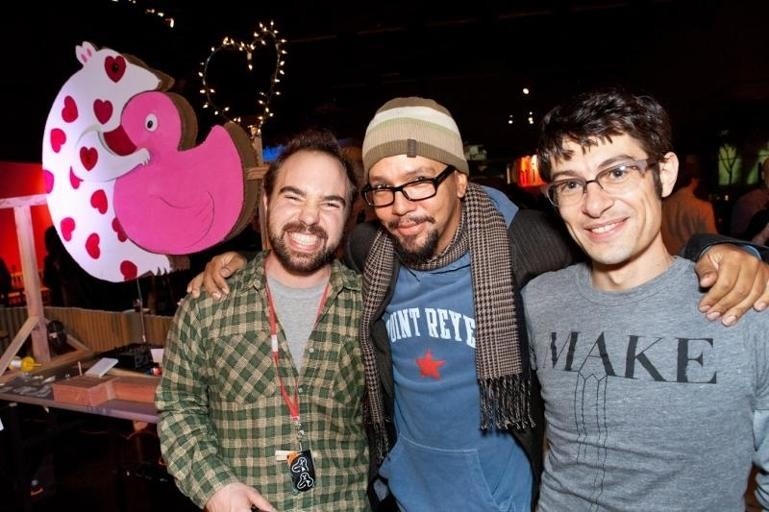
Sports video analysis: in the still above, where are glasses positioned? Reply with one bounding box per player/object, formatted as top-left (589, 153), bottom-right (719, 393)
top-left (545, 157), bottom-right (658, 207)
top-left (360, 164), bottom-right (455, 208)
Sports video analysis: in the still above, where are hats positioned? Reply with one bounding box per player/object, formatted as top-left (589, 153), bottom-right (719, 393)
top-left (363, 97), bottom-right (470, 177)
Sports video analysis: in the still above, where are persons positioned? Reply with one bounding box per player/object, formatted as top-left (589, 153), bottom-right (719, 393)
top-left (659, 153), bottom-right (769, 256)
top-left (154, 129), bottom-right (372, 512)
top-left (186, 96), bottom-right (769, 512)
top-left (42, 223), bottom-right (262, 312)
top-left (520, 92), bottom-right (769, 512)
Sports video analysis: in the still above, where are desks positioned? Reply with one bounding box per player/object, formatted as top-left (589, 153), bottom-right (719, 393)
top-left (0, 367), bottom-right (166, 512)
